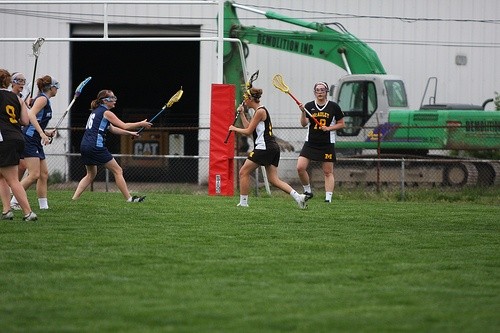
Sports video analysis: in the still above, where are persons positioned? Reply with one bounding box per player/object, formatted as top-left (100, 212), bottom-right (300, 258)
top-left (9, 75), bottom-right (60, 211)
top-left (10, 72), bottom-right (35, 182)
top-left (0, 68), bottom-right (38, 222)
top-left (228, 87), bottom-right (309, 211)
top-left (297, 81), bottom-right (345, 203)
top-left (71, 90), bottom-right (153, 203)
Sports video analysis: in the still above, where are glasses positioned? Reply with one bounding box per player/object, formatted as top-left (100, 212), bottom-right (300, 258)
top-left (14, 79), bottom-right (26, 84)
top-left (43, 81), bottom-right (60, 89)
top-left (315, 88), bottom-right (327, 94)
top-left (102, 95), bottom-right (117, 101)
top-left (242, 95), bottom-right (249, 101)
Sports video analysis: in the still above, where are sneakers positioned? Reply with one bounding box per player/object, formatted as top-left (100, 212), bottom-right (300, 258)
top-left (298, 194), bottom-right (307, 209)
top-left (10, 200), bottom-right (22, 210)
top-left (132, 196), bottom-right (146, 202)
top-left (304, 191), bottom-right (313, 201)
top-left (237, 203), bottom-right (249, 207)
top-left (23, 212), bottom-right (37, 221)
top-left (3, 211), bottom-right (13, 219)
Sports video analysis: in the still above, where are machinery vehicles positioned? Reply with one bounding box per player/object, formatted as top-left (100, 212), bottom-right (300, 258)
top-left (216, 0), bottom-right (500, 188)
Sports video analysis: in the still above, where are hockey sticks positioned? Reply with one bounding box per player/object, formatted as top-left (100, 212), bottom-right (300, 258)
top-left (44, 77), bottom-right (92, 145)
top-left (31, 37), bottom-right (45, 98)
top-left (137, 90), bottom-right (183, 134)
top-left (224, 70), bottom-right (259, 144)
top-left (272, 74), bottom-right (324, 127)
